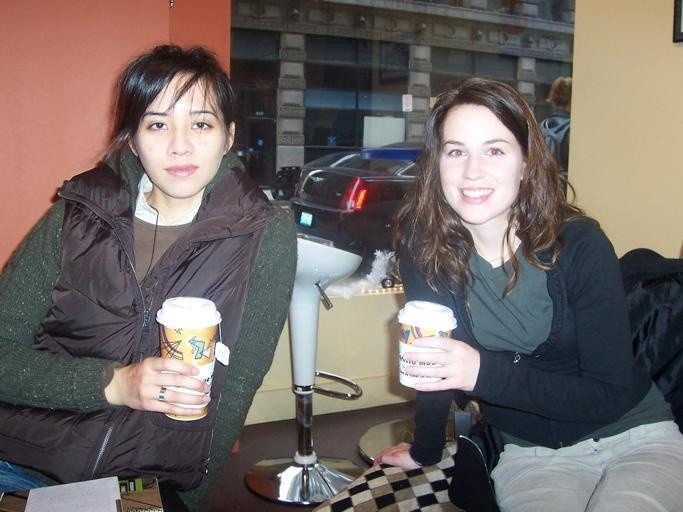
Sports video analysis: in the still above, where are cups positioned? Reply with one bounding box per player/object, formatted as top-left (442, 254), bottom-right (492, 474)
top-left (396, 301), bottom-right (457, 390)
top-left (156, 295), bottom-right (222, 421)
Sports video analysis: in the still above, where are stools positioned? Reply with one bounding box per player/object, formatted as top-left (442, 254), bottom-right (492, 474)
top-left (245, 237), bottom-right (365, 506)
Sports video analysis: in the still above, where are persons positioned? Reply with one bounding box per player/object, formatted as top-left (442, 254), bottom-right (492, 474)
top-left (532, 76), bottom-right (571, 190)
top-left (0, 43), bottom-right (294, 510)
top-left (369, 74), bottom-right (682, 512)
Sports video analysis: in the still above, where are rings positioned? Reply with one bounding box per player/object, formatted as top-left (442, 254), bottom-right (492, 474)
top-left (158, 387), bottom-right (166, 401)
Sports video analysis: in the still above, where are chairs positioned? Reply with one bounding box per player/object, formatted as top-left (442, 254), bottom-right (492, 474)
top-left (312, 248), bottom-right (683, 512)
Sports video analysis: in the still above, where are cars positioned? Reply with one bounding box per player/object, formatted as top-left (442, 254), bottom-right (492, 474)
top-left (291, 143), bottom-right (421, 253)
top-left (236, 145), bottom-right (272, 187)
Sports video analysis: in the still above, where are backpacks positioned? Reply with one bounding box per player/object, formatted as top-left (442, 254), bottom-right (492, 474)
top-left (538, 117), bottom-right (571, 173)
